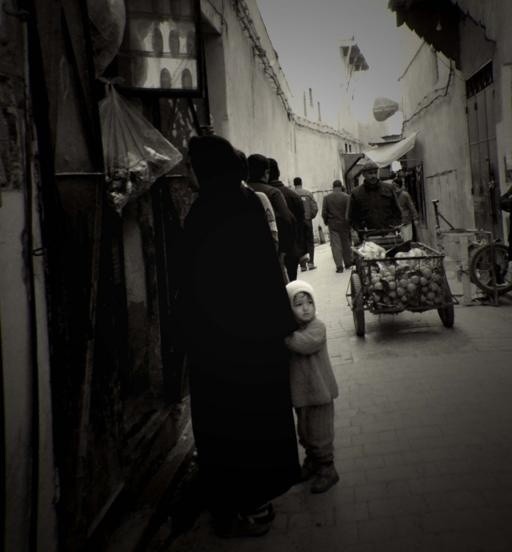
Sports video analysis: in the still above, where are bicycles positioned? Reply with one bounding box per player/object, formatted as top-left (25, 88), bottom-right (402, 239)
top-left (467, 242), bottom-right (512, 294)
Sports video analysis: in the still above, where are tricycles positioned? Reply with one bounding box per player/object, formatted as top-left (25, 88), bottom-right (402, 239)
top-left (343, 221), bottom-right (458, 339)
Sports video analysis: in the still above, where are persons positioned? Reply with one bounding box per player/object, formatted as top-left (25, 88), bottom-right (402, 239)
top-left (185, 137), bottom-right (340, 538)
top-left (322, 161), bottom-right (420, 273)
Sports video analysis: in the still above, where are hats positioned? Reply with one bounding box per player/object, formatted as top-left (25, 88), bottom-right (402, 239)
top-left (360, 154), bottom-right (379, 171)
top-left (285, 280), bottom-right (316, 313)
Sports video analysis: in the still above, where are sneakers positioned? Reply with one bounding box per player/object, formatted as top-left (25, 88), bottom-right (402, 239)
top-left (211, 503), bottom-right (275, 538)
top-left (295, 457), bottom-right (339, 494)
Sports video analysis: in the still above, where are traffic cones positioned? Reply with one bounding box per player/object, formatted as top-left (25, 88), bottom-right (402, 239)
top-left (317, 225), bottom-right (327, 245)
